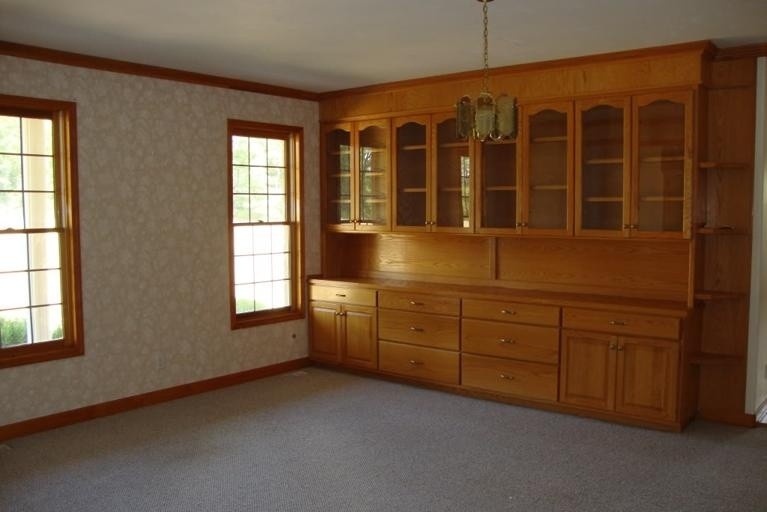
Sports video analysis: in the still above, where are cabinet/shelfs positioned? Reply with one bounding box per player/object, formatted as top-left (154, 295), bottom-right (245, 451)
top-left (692, 59), bottom-right (757, 430)
top-left (321, 85), bottom-right (392, 232)
top-left (575, 51), bottom-right (694, 241)
top-left (376, 290), bottom-right (560, 406)
top-left (559, 306), bottom-right (701, 432)
top-left (391, 71), bottom-right (475, 236)
top-left (307, 284), bottom-right (377, 372)
top-left (474, 59), bottom-right (575, 237)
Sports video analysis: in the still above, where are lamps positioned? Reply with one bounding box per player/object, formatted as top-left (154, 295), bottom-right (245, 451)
top-left (454, 0), bottom-right (517, 145)
